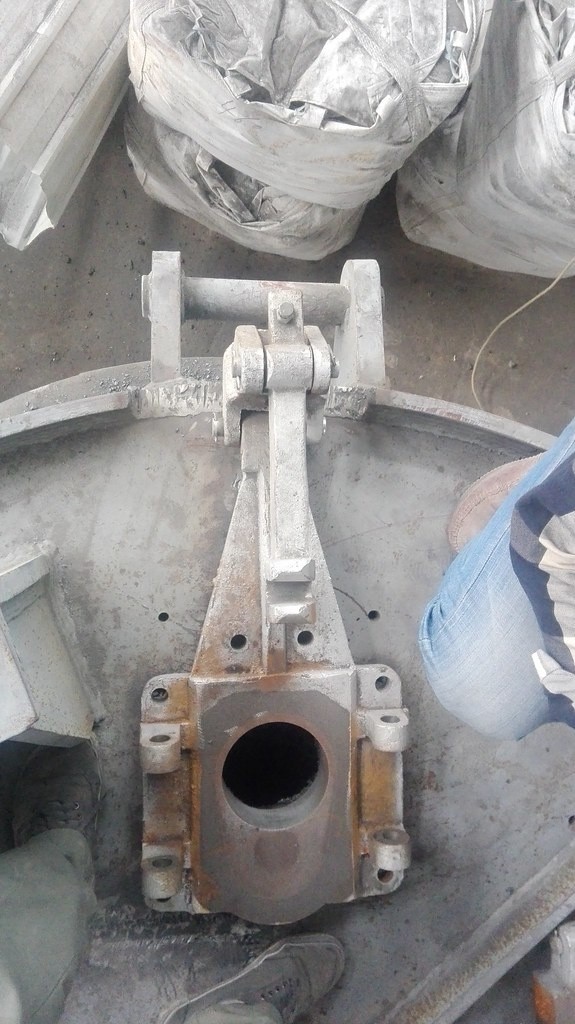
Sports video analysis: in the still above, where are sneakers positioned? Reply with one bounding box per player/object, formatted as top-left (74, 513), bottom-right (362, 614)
top-left (158, 933), bottom-right (345, 1024)
top-left (13, 732), bottom-right (103, 860)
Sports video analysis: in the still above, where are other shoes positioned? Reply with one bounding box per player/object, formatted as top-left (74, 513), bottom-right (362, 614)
top-left (449, 450), bottom-right (549, 554)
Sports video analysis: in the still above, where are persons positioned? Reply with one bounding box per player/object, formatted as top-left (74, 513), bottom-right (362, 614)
top-left (419, 416), bottom-right (575, 740)
top-left (1, 730), bottom-right (346, 1023)
